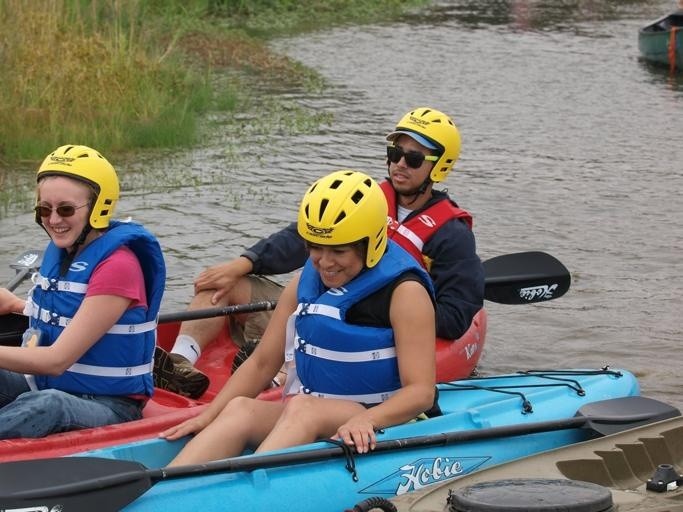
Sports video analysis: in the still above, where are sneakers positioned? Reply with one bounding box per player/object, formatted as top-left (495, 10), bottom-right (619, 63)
top-left (152, 346), bottom-right (210, 401)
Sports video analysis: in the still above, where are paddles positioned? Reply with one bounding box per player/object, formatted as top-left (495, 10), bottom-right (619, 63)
top-left (5, 250), bottom-right (46, 292)
top-left (0, 398), bottom-right (681, 511)
top-left (157, 252), bottom-right (572, 322)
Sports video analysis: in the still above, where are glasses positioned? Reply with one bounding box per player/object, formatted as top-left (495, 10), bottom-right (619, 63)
top-left (34, 202), bottom-right (91, 219)
top-left (387, 146), bottom-right (437, 169)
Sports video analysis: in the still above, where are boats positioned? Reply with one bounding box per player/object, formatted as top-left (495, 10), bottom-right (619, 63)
top-left (634, 8), bottom-right (681, 77)
top-left (59, 364), bottom-right (643, 509)
top-left (352, 410), bottom-right (683, 512)
top-left (1, 291), bottom-right (487, 471)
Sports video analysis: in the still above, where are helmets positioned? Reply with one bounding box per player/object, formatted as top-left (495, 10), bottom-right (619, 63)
top-left (36, 144), bottom-right (120, 229)
top-left (296, 170), bottom-right (388, 269)
top-left (396, 107), bottom-right (462, 183)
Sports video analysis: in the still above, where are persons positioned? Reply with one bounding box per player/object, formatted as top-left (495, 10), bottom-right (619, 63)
top-left (153, 106), bottom-right (486, 397)
top-left (0, 143), bottom-right (165, 440)
top-left (158, 170), bottom-right (436, 473)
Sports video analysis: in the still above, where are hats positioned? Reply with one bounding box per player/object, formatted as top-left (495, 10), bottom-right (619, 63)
top-left (386, 131), bottom-right (437, 152)
